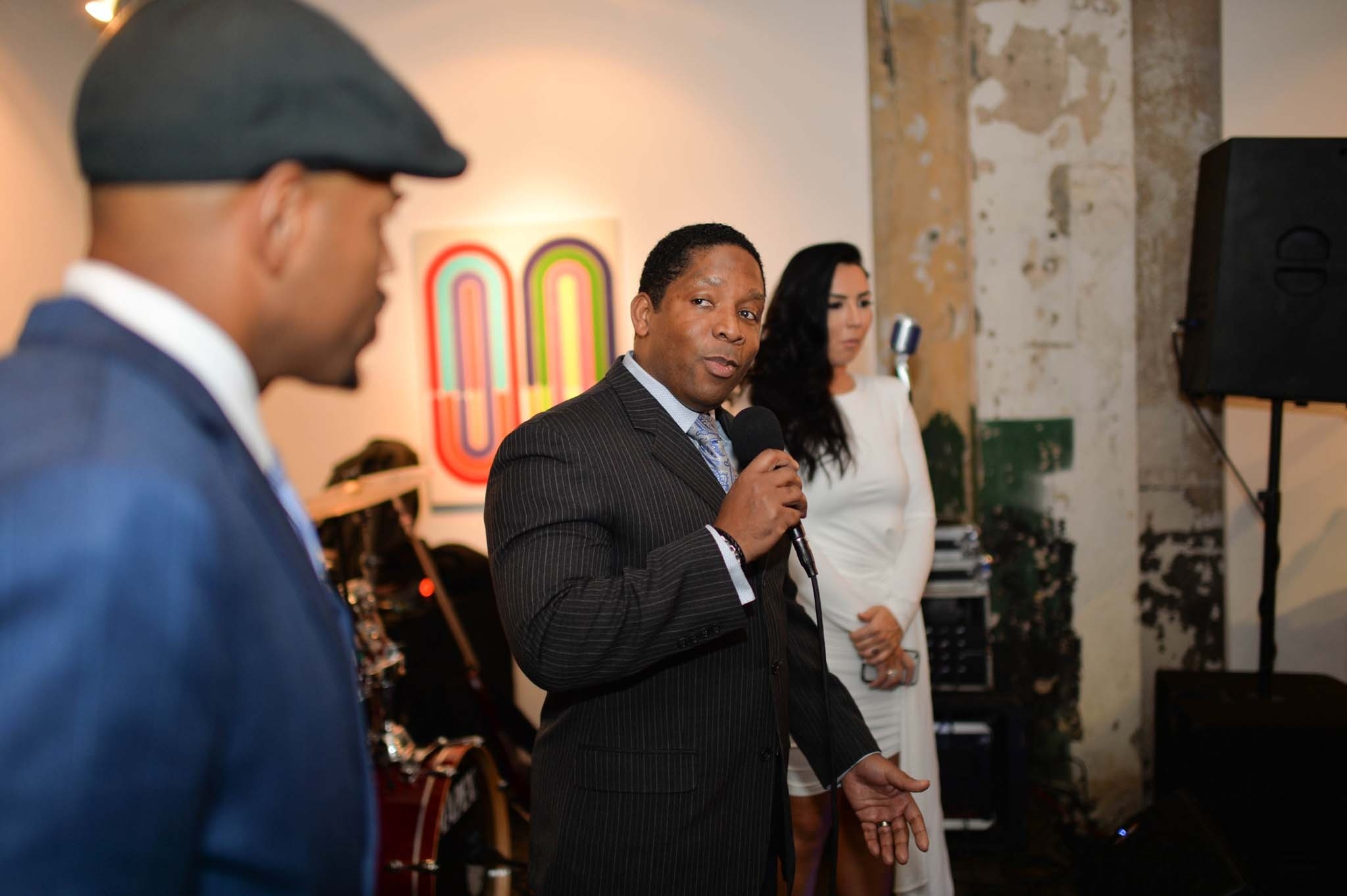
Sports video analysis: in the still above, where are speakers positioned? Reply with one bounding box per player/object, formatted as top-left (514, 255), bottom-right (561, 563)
top-left (1142, 670), bottom-right (1347, 896)
top-left (1179, 136), bottom-right (1347, 404)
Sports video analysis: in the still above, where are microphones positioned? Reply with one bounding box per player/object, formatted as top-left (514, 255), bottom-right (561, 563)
top-left (732, 405), bottom-right (817, 577)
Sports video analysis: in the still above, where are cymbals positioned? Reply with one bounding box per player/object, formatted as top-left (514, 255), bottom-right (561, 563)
top-left (304, 463), bottom-right (434, 522)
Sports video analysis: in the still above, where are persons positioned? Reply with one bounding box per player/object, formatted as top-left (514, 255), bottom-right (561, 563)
top-left (333, 225), bottom-right (960, 896)
top-left (1, 0), bottom-right (473, 895)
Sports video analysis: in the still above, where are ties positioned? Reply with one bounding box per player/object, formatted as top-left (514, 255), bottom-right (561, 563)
top-left (687, 412), bottom-right (739, 495)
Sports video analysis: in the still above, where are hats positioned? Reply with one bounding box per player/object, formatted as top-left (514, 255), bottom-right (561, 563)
top-left (76, 1), bottom-right (468, 181)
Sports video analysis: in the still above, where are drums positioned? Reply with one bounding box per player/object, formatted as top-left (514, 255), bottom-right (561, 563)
top-left (334, 579), bottom-right (404, 684)
top-left (373, 736), bottom-right (511, 896)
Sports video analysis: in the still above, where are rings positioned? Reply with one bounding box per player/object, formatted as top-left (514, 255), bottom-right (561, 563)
top-left (877, 821), bottom-right (892, 827)
top-left (886, 669), bottom-right (896, 679)
top-left (871, 647), bottom-right (879, 656)
